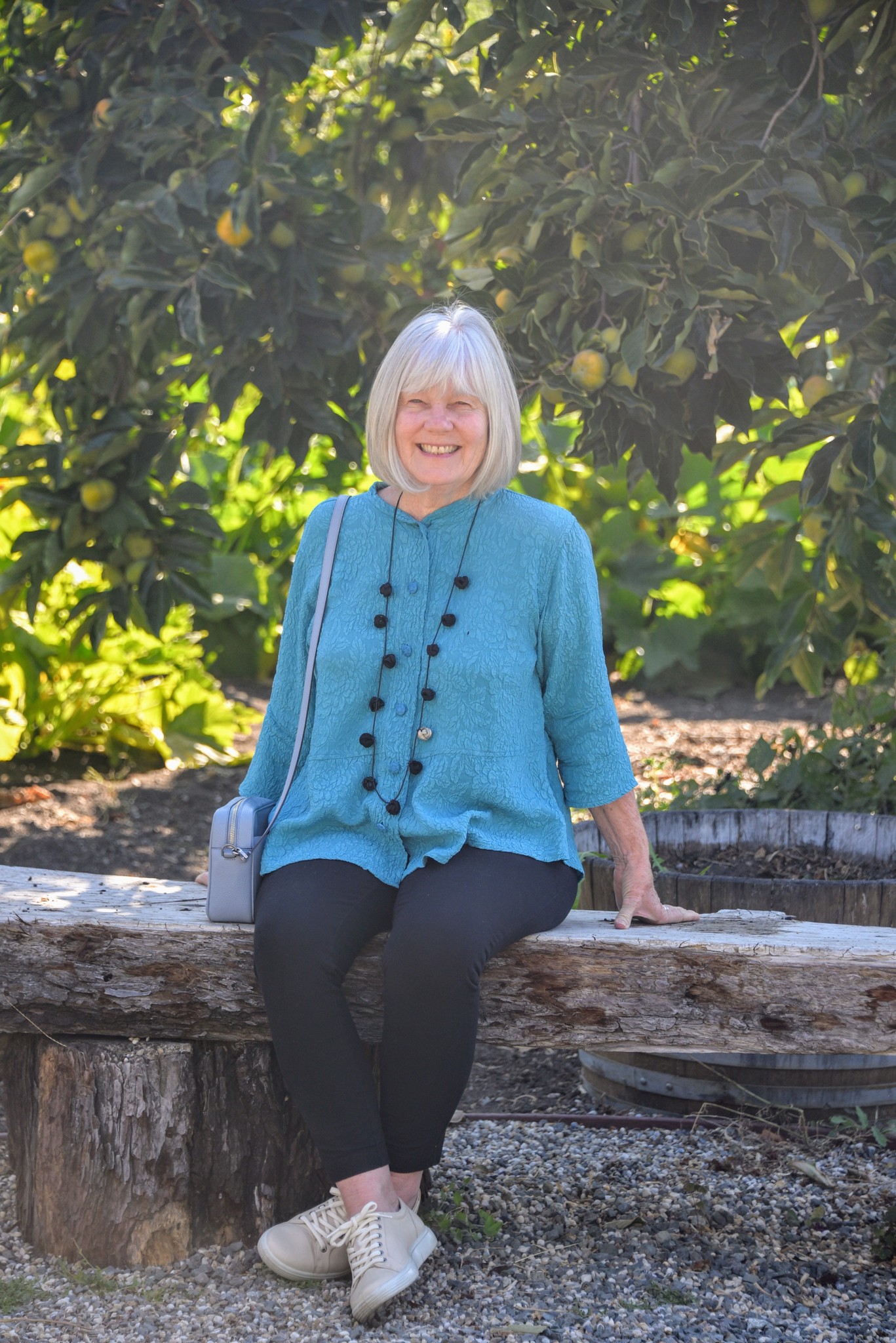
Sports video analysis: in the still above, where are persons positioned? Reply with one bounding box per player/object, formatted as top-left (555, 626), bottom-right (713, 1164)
top-left (239, 305), bottom-right (700, 1318)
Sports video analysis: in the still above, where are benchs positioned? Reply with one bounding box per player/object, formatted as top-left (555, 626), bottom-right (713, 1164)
top-left (0, 875), bottom-right (896, 1269)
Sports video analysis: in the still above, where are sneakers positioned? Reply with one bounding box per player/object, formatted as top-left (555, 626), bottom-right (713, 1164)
top-left (257, 1186), bottom-right (422, 1281)
top-left (326, 1196), bottom-right (438, 1323)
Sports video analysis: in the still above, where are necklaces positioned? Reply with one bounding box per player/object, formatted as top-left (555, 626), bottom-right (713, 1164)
top-left (360, 492), bottom-right (482, 816)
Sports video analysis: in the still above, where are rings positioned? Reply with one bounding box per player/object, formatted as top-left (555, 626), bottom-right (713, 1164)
top-left (663, 906), bottom-right (669, 910)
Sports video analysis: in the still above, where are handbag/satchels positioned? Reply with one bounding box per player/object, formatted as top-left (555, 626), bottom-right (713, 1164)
top-left (205, 795), bottom-right (277, 924)
